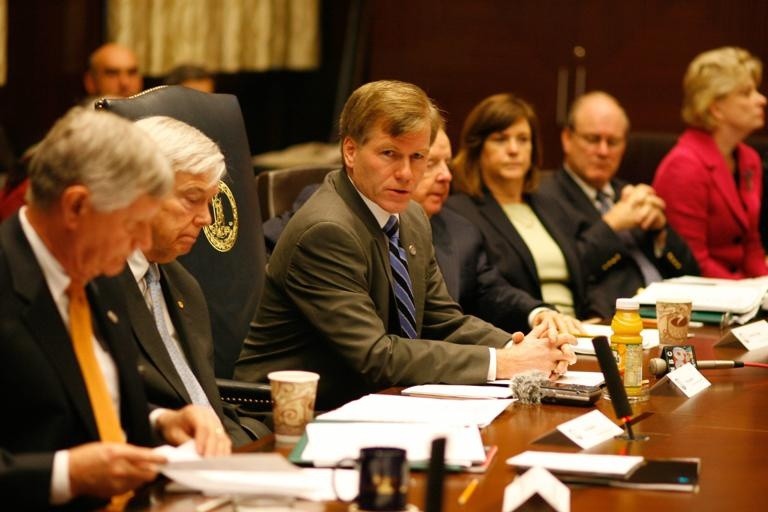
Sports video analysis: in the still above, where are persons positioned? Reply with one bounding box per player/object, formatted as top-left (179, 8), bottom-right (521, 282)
top-left (539, 92), bottom-right (700, 320)
top-left (85, 44), bottom-right (141, 97)
top-left (410, 128), bottom-right (584, 335)
top-left (167, 66), bottom-right (215, 94)
top-left (444, 93), bottom-right (615, 324)
top-left (109, 116), bottom-right (274, 451)
top-left (0, 106), bottom-right (232, 512)
top-left (653, 47), bottom-right (768, 280)
top-left (234, 80), bottom-right (578, 410)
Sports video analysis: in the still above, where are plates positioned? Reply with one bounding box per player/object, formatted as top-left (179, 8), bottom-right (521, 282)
top-left (347, 503), bottom-right (422, 512)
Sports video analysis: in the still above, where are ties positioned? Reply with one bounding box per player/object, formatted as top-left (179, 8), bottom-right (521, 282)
top-left (65, 279), bottom-right (129, 448)
top-left (380, 215), bottom-right (420, 342)
top-left (144, 265), bottom-right (211, 404)
top-left (596, 190), bottom-right (663, 287)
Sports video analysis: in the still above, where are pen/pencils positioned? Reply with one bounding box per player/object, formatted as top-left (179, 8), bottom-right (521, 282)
top-left (457, 478), bottom-right (480, 504)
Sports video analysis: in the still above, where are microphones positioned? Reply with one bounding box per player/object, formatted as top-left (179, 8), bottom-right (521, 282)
top-left (592, 336), bottom-right (635, 438)
top-left (647, 358), bottom-right (744, 376)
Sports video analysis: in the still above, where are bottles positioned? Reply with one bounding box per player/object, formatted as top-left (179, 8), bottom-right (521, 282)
top-left (608, 296), bottom-right (642, 397)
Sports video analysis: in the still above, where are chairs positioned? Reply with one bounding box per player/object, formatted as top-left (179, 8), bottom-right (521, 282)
top-left (94, 85), bottom-right (320, 445)
top-left (256, 162), bottom-right (339, 251)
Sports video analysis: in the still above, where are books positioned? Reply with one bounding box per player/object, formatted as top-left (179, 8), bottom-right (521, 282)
top-left (633, 276), bottom-right (768, 327)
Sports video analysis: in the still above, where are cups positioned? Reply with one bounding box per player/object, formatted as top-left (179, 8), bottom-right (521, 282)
top-left (332, 447), bottom-right (409, 511)
top-left (266, 369), bottom-right (321, 444)
top-left (656, 295), bottom-right (693, 349)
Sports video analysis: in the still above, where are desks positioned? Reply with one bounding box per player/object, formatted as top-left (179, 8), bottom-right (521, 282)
top-left (145, 309), bottom-right (768, 511)
